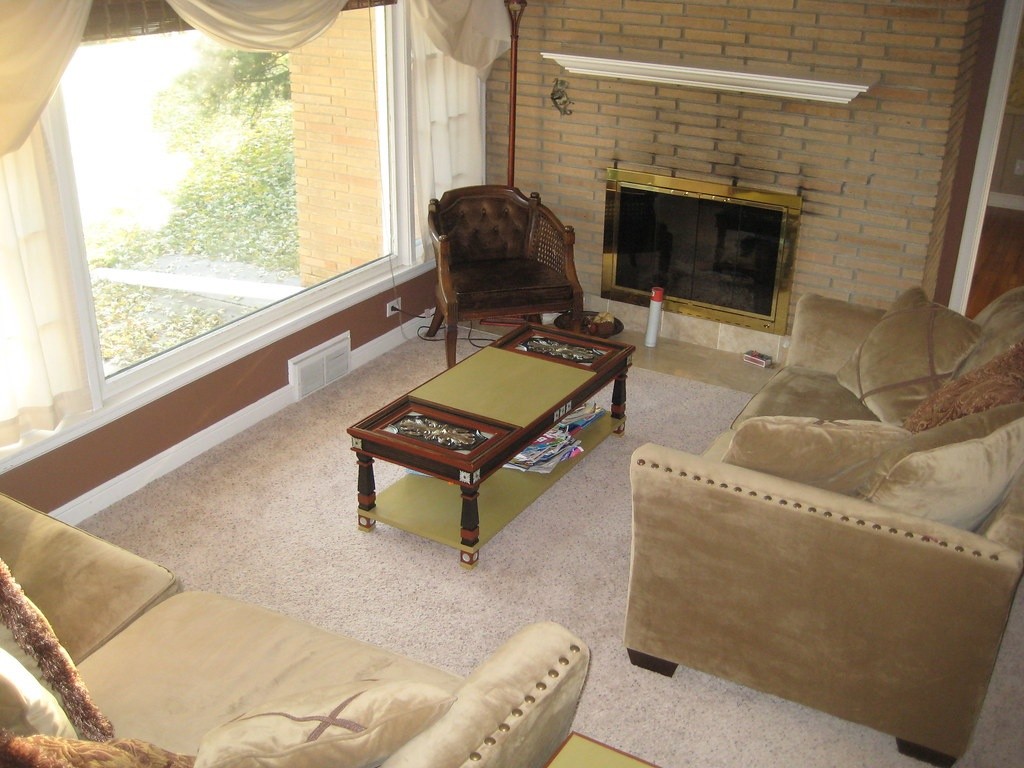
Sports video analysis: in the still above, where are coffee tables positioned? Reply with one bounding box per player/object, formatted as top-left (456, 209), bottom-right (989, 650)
top-left (713, 209), bottom-right (782, 279)
top-left (347, 322), bottom-right (637, 571)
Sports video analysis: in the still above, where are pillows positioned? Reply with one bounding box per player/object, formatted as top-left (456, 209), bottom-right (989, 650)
top-left (865, 416), bottom-right (1024, 530)
top-left (720, 416), bottom-right (911, 495)
top-left (1, 556), bottom-right (113, 743)
top-left (903, 342), bottom-right (1024, 433)
top-left (1, 649), bottom-right (76, 740)
top-left (870, 403), bottom-right (1024, 491)
top-left (2, 726), bottom-right (196, 768)
top-left (194, 679), bottom-right (459, 768)
top-left (836, 286), bottom-right (983, 428)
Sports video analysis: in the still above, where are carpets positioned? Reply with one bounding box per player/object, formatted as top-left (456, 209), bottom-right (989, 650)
top-left (544, 731), bottom-right (658, 768)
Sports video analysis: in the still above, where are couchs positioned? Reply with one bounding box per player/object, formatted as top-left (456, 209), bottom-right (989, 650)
top-left (624, 288), bottom-right (1024, 768)
top-left (1, 491), bottom-right (593, 767)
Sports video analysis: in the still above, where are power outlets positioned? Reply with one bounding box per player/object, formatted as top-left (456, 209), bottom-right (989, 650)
top-left (386, 297), bottom-right (402, 317)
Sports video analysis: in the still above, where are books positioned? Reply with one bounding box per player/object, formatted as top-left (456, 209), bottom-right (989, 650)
top-left (552, 407), bottom-right (607, 436)
top-left (502, 404), bottom-right (605, 474)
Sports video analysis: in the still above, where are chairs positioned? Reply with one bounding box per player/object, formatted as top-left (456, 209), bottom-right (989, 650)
top-left (425, 185), bottom-right (583, 369)
top-left (618, 192), bottom-right (672, 271)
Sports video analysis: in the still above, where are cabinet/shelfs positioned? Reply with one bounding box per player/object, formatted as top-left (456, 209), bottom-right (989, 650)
top-left (987, 113), bottom-right (1024, 212)
